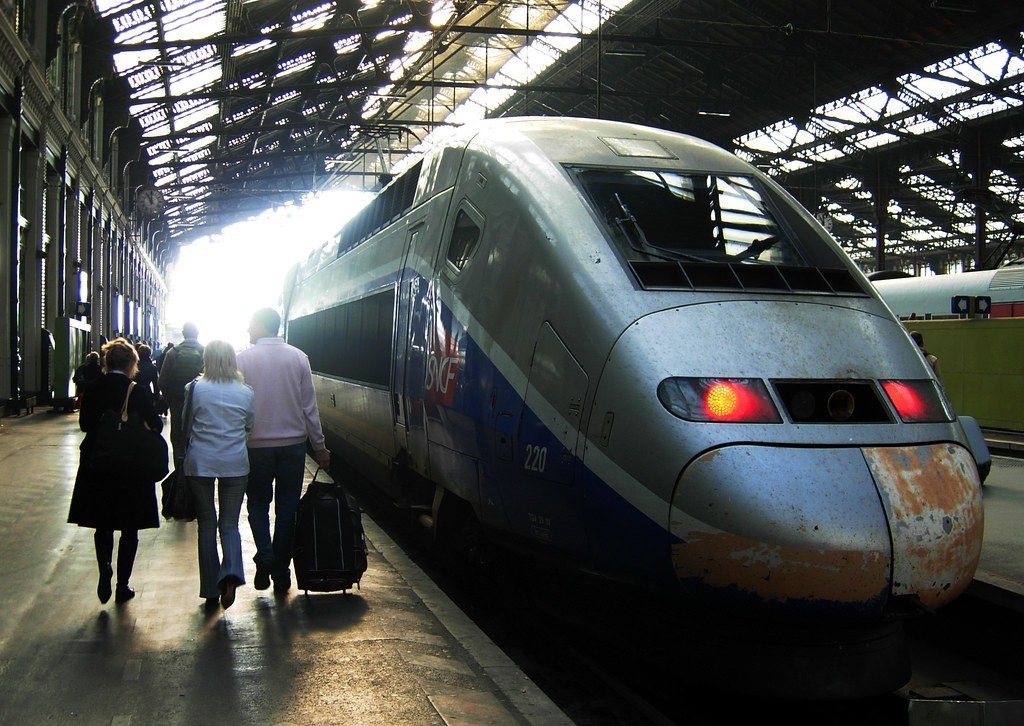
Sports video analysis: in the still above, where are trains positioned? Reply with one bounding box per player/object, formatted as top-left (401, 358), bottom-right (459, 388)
top-left (254, 115), bottom-right (986, 628)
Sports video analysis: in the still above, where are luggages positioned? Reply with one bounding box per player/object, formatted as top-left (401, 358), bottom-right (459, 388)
top-left (294, 466), bottom-right (369, 596)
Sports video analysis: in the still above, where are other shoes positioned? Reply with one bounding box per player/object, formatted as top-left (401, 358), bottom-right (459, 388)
top-left (254, 558), bottom-right (271, 590)
top-left (220, 575), bottom-right (236, 610)
top-left (206, 597), bottom-right (219, 609)
top-left (273, 576), bottom-right (290, 595)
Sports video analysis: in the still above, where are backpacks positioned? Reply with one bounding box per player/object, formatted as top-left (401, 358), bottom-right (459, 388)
top-left (172, 342), bottom-right (204, 398)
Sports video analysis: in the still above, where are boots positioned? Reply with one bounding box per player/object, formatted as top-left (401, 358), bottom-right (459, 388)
top-left (95, 533), bottom-right (113, 604)
top-left (115, 537), bottom-right (138, 604)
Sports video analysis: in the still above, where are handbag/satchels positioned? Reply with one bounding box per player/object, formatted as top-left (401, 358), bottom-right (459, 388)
top-left (161, 468), bottom-right (197, 522)
top-left (79, 381), bottom-right (169, 482)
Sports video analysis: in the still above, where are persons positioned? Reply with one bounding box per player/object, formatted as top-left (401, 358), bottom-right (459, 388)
top-left (72, 322), bottom-right (206, 469)
top-left (235, 307), bottom-right (330, 595)
top-left (66, 337), bottom-right (163, 604)
top-left (181, 339), bottom-right (255, 612)
top-left (910, 331), bottom-right (940, 382)
